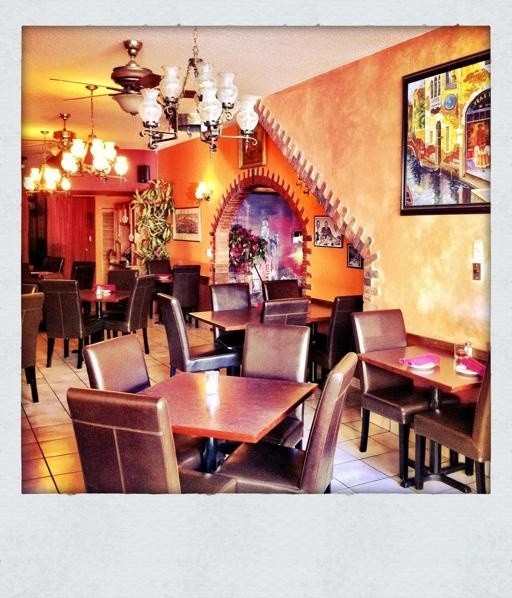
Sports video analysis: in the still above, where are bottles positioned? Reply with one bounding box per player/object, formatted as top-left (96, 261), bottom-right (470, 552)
top-left (97, 294), bottom-right (102, 300)
top-left (457, 341), bottom-right (472, 361)
top-left (97, 286), bottom-right (102, 295)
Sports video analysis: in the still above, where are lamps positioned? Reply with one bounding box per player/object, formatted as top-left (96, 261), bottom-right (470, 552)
top-left (21, 129), bottom-right (73, 196)
top-left (135, 25), bottom-right (261, 157)
top-left (112, 92), bottom-right (147, 115)
top-left (194, 180), bottom-right (212, 202)
top-left (58, 82), bottom-right (130, 185)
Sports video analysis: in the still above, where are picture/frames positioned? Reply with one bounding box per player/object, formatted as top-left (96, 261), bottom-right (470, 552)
top-left (347, 243), bottom-right (361, 270)
top-left (313, 214), bottom-right (344, 248)
top-left (172, 206), bottom-right (201, 243)
top-left (399, 49), bottom-right (489, 217)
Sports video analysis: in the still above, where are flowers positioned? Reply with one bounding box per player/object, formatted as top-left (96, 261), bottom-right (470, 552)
top-left (228, 225), bottom-right (268, 274)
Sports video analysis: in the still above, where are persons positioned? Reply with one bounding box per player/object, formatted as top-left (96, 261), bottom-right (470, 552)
top-left (469, 123), bottom-right (491, 159)
top-left (320, 219), bottom-right (334, 239)
top-left (316, 218), bottom-right (321, 241)
top-left (141, 239), bottom-right (147, 247)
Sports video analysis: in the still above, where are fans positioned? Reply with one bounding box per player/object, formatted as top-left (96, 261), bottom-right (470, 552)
top-left (49, 39), bottom-right (197, 103)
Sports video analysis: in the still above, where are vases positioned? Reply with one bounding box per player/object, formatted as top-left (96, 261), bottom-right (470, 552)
top-left (237, 270), bottom-right (254, 296)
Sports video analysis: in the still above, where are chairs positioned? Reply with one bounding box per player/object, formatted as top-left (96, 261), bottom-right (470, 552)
top-left (20, 253), bottom-right (492, 493)
